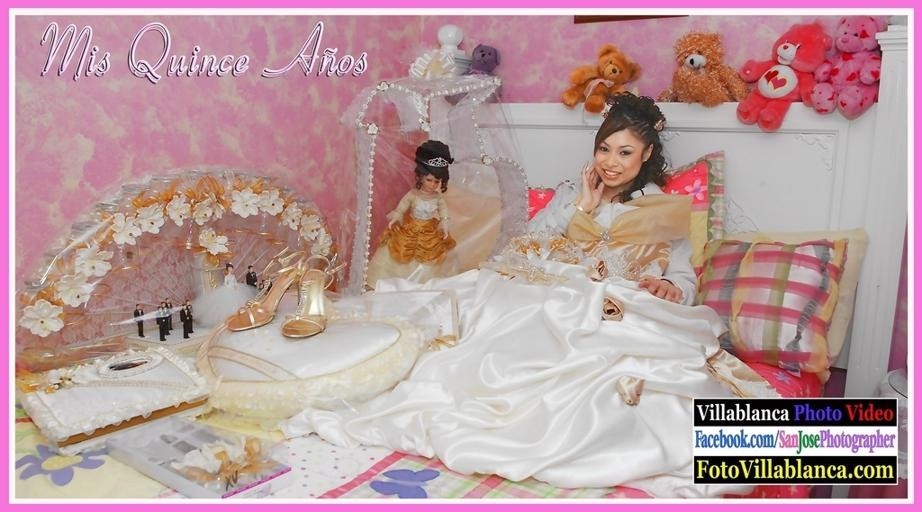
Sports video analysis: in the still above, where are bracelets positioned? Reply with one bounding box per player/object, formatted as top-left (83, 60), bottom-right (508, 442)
top-left (570, 200), bottom-right (585, 214)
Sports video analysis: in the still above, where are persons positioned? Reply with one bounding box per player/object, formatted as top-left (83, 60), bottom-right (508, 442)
top-left (498, 90), bottom-right (701, 305)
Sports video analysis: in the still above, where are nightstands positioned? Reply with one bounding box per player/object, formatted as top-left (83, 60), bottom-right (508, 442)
top-left (847, 369), bottom-right (909, 499)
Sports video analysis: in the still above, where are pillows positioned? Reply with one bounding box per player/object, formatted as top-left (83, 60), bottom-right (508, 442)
top-left (694, 232), bottom-right (862, 381)
top-left (529, 149), bottom-right (725, 284)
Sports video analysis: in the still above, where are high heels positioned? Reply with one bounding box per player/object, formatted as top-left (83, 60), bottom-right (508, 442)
top-left (282, 253), bottom-right (336, 341)
top-left (224, 248), bottom-right (310, 332)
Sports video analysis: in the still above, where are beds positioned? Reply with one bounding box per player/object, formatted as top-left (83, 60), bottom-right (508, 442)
top-left (16, 21), bottom-right (910, 499)
